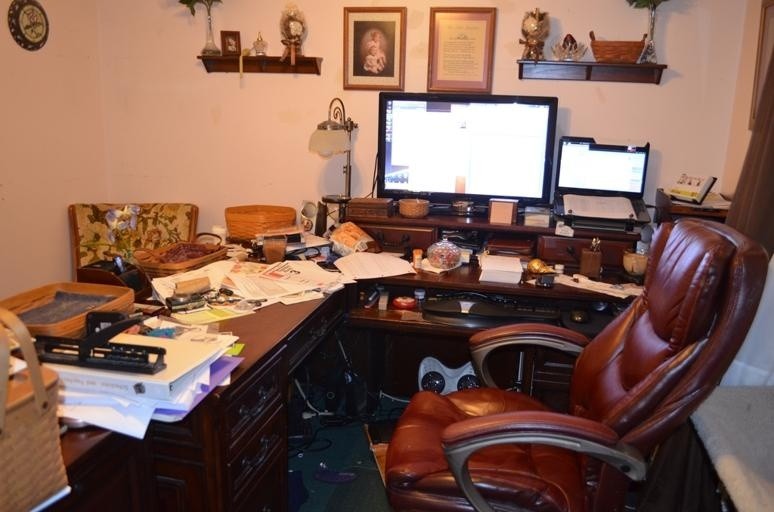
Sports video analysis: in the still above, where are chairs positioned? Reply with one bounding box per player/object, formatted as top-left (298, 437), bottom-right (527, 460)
top-left (387, 215), bottom-right (771, 510)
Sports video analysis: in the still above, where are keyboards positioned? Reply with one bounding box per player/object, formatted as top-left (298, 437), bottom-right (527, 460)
top-left (426, 294), bottom-right (562, 331)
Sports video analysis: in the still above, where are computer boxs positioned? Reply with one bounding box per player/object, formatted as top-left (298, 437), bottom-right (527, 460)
top-left (306, 325), bottom-right (382, 414)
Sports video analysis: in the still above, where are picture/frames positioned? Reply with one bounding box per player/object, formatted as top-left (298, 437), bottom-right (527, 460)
top-left (219, 28), bottom-right (242, 56)
top-left (341, 4), bottom-right (408, 91)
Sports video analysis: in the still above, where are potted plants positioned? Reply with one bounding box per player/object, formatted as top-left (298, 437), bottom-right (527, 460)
top-left (179, 0), bottom-right (221, 56)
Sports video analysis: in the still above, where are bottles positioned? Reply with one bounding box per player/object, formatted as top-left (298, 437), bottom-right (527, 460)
top-left (413, 249), bottom-right (423, 272)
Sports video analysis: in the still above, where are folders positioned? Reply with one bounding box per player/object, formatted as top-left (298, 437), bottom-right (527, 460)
top-left (42, 332), bottom-right (224, 400)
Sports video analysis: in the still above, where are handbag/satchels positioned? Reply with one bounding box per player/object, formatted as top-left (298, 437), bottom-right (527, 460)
top-left (0, 308), bottom-right (69, 512)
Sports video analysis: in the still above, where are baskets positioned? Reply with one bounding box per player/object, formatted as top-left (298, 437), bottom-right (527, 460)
top-left (0, 281), bottom-right (135, 340)
top-left (590, 31), bottom-right (647, 64)
top-left (132, 232), bottom-right (229, 283)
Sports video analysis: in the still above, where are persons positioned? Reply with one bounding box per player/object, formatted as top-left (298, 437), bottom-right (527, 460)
top-left (363, 46), bottom-right (383, 75)
top-left (366, 31), bottom-right (387, 68)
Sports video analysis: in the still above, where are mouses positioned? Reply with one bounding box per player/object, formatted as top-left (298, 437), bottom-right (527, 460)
top-left (571, 306), bottom-right (589, 323)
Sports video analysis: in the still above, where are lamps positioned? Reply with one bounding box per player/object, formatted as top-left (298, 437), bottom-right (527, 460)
top-left (308, 98), bottom-right (358, 206)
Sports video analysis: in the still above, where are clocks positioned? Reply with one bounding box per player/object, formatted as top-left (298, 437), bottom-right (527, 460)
top-left (7, 0), bottom-right (49, 53)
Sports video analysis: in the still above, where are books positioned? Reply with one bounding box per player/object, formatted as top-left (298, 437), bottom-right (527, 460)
top-left (478, 254), bottom-right (524, 286)
top-left (668, 172), bottom-right (714, 204)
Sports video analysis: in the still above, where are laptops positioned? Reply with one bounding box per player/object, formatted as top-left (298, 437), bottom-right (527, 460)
top-left (554, 135), bottom-right (652, 225)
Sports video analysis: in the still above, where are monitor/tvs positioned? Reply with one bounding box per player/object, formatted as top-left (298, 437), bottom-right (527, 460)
top-left (377, 91), bottom-right (558, 215)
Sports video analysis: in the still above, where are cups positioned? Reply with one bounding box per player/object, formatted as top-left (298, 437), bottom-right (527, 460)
top-left (623, 254), bottom-right (648, 275)
top-left (262, 232), bottom-right (288, 263)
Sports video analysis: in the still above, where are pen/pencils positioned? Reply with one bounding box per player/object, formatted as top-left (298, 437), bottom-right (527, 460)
top-left (369, 291), bottom-right (377, 301)
top-left (590, 237), bottom-right (601, 252)
top-left (521, 272), bottom-right (524, 284)
